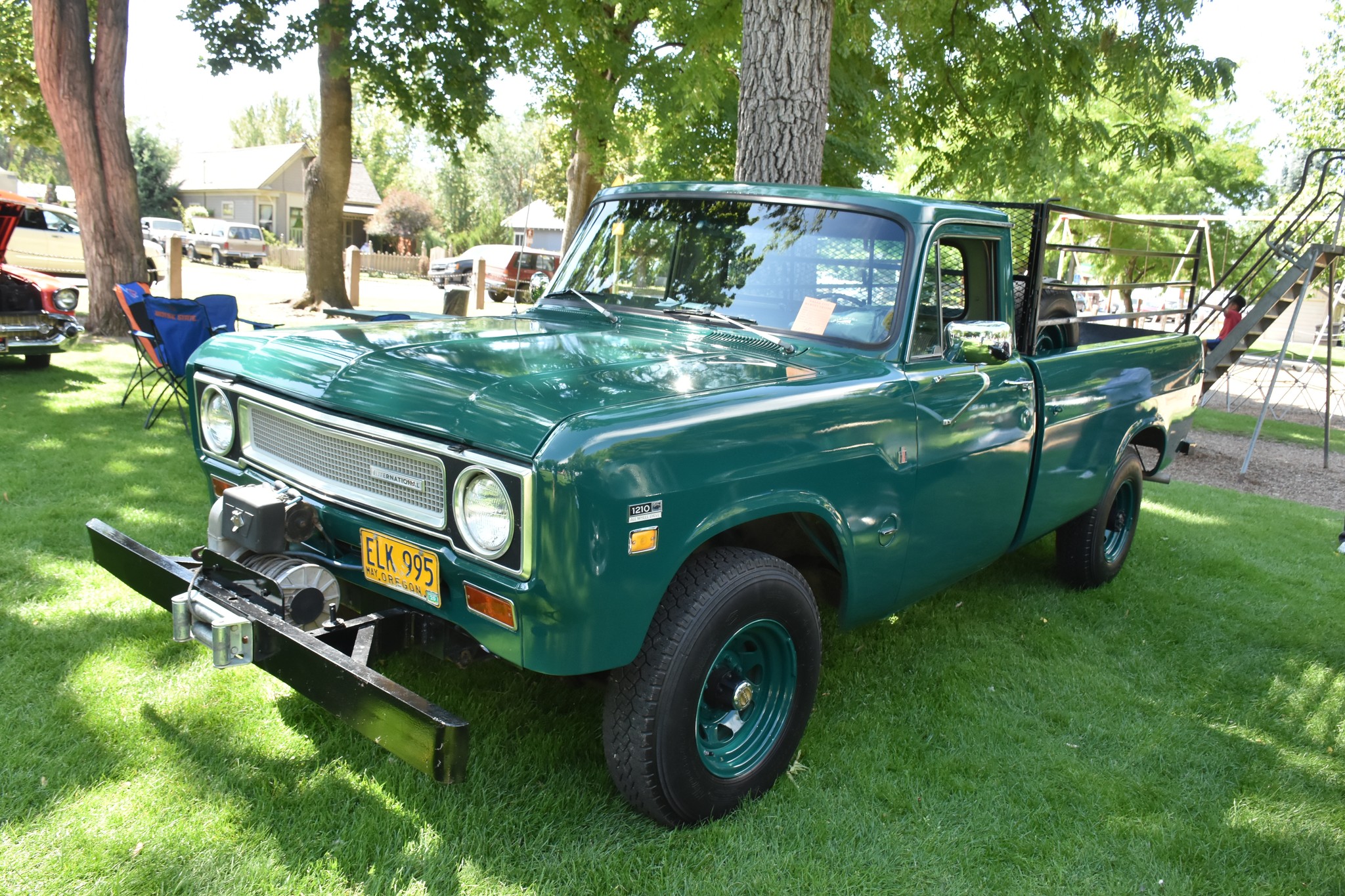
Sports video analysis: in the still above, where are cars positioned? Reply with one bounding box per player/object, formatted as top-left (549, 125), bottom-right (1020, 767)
top-left (428, 244), bottom-right (560, 304)
top-left (0, 191), bottom-right (168, 367)
top-left (139, 216), bottom-right (268, 269)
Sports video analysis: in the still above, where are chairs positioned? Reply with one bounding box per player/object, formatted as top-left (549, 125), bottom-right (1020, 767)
top-left (112, 281), bottom-right (285, 437)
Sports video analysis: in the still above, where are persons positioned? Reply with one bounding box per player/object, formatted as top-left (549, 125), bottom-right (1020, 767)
top-left (1200, 295), bottom-right (1247, 356)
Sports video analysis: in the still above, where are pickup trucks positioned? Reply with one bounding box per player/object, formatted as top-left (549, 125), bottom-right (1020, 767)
top-left (85, 182), bottom-right (1205, 833)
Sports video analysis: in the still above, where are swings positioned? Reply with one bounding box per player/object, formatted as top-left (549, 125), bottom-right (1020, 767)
top-left (1217, 222), bottom-right (1264, 306)
top-left (1082, 219), bottom-right (1113, 285)
top-left (1142, 220), bottom-right (1184, 283)
